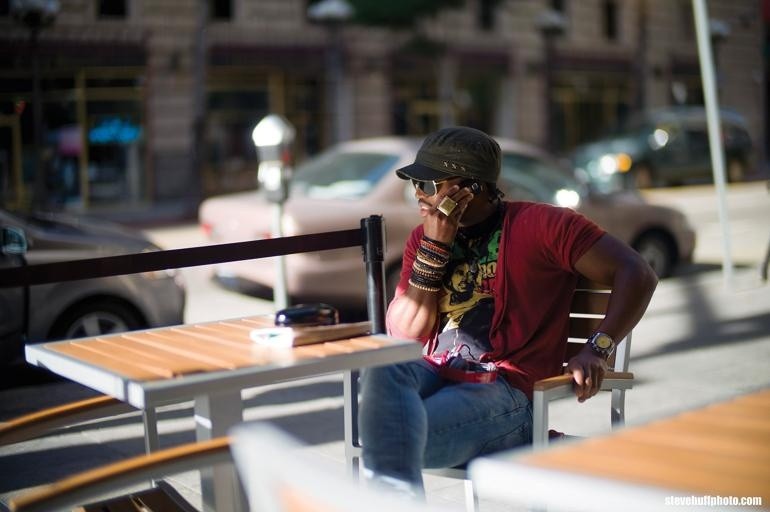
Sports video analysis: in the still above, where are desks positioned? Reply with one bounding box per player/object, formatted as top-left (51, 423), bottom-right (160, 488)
top-left (467, 377), bottom-right (769, 508)
top-left (20, 313), bottom-right (421, 511)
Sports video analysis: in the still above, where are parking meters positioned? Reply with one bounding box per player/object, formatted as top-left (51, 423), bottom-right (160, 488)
top-left (251, 113), bottom-right (296, 313)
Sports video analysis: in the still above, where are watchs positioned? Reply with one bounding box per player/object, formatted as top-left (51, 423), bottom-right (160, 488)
top-left (588, 330), bottom-right (617, 361)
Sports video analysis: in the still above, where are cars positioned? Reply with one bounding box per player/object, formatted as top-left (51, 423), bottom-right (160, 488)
top-left (2, 203), bottom-right (191, 380)
top-left (568, 105), bottom-right (768, 272)
top-left (197, 134), bottom-right (698, 322)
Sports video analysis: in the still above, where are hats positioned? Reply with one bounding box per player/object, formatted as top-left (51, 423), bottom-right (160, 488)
top-left (395, 126), bottom-right (501, 183)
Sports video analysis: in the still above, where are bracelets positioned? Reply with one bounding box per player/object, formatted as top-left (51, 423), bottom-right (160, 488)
top-left (407, 236), bottom-right (453, 293)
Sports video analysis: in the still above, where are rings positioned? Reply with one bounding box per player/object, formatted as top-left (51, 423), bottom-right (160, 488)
top-left (437, 195), bottom-right (458, 216)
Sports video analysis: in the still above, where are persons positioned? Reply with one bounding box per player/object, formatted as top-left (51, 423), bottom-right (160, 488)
top-left (358, 125), bottom-right (659, 503)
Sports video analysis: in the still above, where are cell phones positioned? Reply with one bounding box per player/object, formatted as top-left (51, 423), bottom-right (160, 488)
top-left (458, 177), bottom-right (482, 195)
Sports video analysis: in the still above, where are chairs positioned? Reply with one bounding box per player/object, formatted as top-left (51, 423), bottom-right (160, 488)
top-left (534, 260), bottom-right (639, 447)
top-left (1, 385), bottom-right (231, 512)
top-left (225, 418), bottom-right (438, 511)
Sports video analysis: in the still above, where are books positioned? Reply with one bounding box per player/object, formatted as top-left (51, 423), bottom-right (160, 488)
top-left (251, 321), bottom-right (374, 349)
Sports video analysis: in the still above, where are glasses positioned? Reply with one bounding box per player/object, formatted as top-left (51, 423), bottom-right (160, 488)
top-left (412, 177), bottom-right (450, 196)
